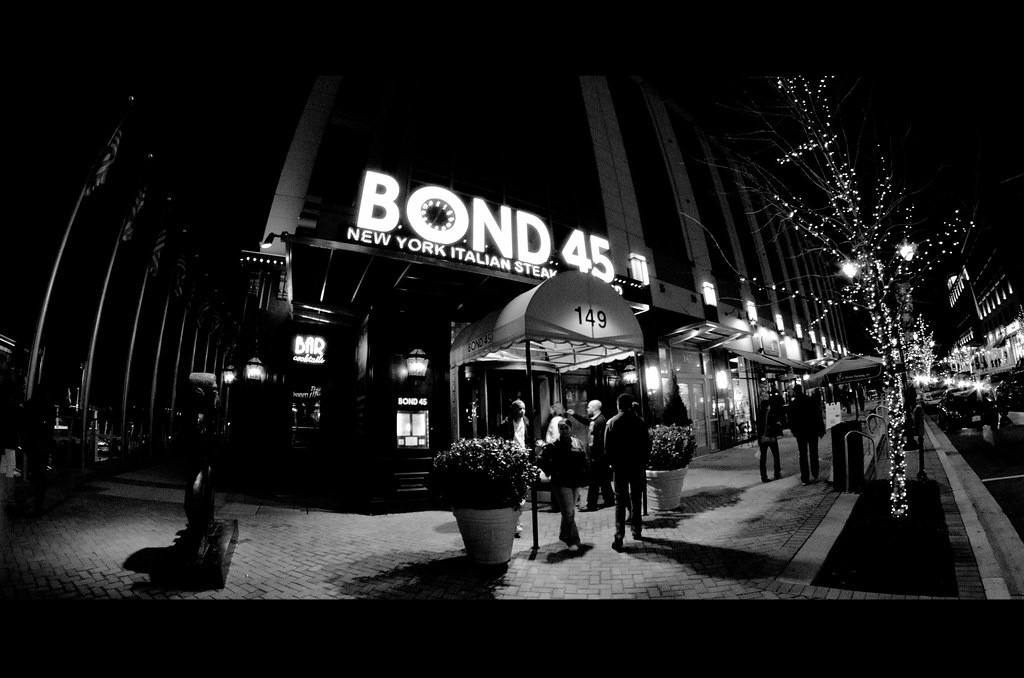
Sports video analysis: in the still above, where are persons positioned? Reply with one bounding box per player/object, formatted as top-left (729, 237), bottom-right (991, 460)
top-left (604, 394), bottom-right (650, 550)
top-left (534, 403), bottom-right (590, 553)
top-left (756, 384), bottom-right (825, 485)
top-left (991, 357), bottom-right (1001, 367)
top-left (567, 399), bottom-right (615, 511)
top-left (826, 377), bottom-right (882, 415)
top-left (495, 399), bottom-right (529, 534)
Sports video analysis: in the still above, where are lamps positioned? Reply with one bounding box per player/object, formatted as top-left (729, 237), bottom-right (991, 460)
top-left (264, 233), bottom-right (286, 243)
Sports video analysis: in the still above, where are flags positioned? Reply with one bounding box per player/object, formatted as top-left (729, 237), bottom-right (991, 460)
top-left (122, 183), bottom-right (147, 240)
top-left (175, 253), bottom-right (186, 296)
top-left (149, 227), bottom-right (166, 277)
top-left (85, 125), bottom-right (122, 195)
top-left (186, 283), bottom-right (224, 346)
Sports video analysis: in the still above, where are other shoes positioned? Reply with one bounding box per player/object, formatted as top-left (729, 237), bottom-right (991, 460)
top-left (801, 478), bottom-right (811, 486)
top-left (612, 538), bottom-right (623, 551)
top-left (625, 517), bottom-right (632, 525)
top-left (774, 474), bottom-right (782, 480)
top-left (578, 505), bottom-right (597, 511)
top-left (569, 544), bottom-right (578, 551)
top-left (632, 530), bottom-right (642, 540)
top-left (761, 476), bottom-right (770, 483)
top-left (812, 474), bottom-right (819, 479)
top-left (600, 501), bottom-right (615, 506)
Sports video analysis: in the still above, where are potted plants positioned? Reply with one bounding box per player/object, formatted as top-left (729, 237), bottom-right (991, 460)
top-left (430, 436), bottom-right (540, 564)
top-left (647, 423), bottom-right (698, 512)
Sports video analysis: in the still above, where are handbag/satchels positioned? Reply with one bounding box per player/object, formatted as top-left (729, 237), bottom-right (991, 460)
top-left (760, 435), bottom-right (776, 445)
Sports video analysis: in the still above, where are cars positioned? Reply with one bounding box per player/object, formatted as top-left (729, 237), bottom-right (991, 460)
top-left (868, 390), bottom-right (877, 398)
top-left (916, 364), bottom-right (1024, 435)
top-left (936, 386), bottom-right (999, 437)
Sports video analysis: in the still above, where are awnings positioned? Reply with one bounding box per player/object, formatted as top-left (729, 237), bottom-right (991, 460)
top-left (788, 359), bottom-right (821, 370)
top-left (762, 355), bottom-right (808, 369)
top-left (451, 270), bottom-right (648, 552)
top-left (725, 347), bottom-right (786, 367)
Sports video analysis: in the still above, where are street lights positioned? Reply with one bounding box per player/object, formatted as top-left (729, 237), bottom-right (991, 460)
top-left (832, 232), bottom-right (920, 449)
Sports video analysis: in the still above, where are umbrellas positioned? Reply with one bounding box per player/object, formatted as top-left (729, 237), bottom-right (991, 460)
top-left (808, 355), bottom-right (883, 420)
